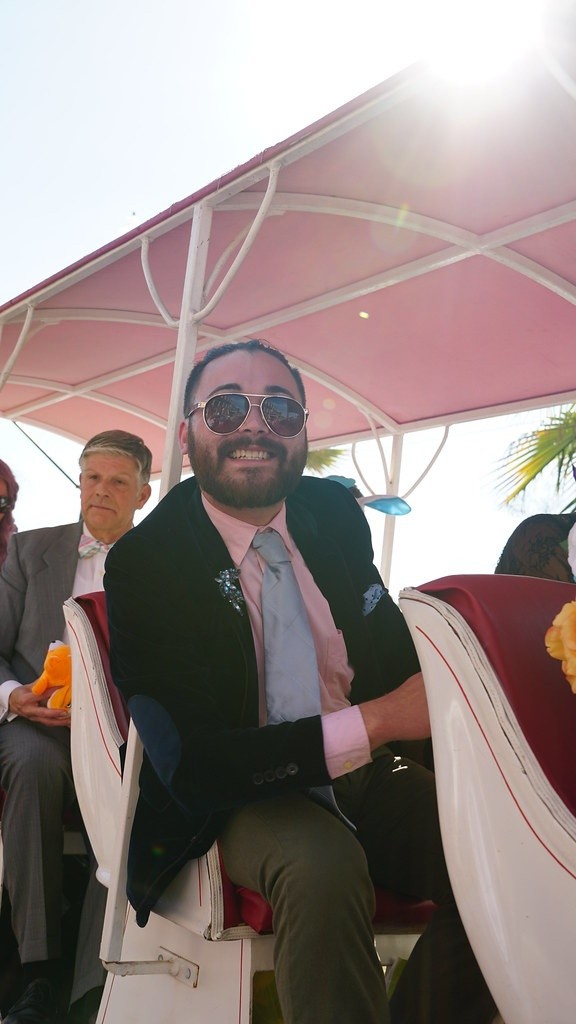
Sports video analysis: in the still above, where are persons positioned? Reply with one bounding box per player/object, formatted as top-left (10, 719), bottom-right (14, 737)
top-left (0, 460), bottom-right (19, 565)
top-left (494, 513), bottom-right (576, 582)
top-left (0, 430), bottom-right (153, 1024)
top-left (102, 337), bottom-right (500, 1024)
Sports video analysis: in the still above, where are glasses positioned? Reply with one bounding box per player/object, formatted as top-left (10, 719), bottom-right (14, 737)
top-left (185, 393), bottom-right (309, 439)
top-left (0, 496), bottom-right (11, 511)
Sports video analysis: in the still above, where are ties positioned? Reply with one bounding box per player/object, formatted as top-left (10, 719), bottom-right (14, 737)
top-left (250, 529), bottom-right (356, 832)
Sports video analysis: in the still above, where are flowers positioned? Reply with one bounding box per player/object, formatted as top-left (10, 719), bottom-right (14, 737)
top-left (544, 600), bottom-right (576, 694)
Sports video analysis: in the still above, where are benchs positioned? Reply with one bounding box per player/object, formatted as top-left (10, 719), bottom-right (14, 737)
top-left (398, 572), bottom-right (576, 1024)
top-left (63, 590), bottom-right (441, 1024)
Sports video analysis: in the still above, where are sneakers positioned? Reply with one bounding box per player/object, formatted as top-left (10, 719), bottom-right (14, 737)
top-left (2, 978), bottom-right (57, 1024)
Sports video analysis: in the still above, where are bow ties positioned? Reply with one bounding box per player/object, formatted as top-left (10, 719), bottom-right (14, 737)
top-left (78, 535), bottom-right (109, 559)
